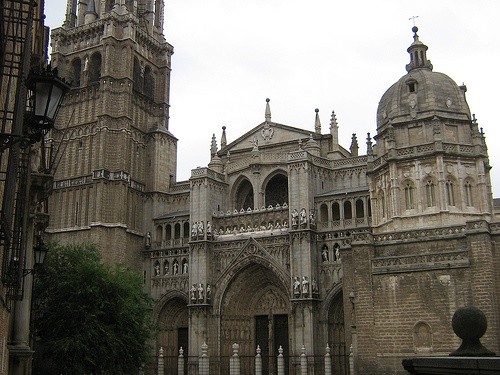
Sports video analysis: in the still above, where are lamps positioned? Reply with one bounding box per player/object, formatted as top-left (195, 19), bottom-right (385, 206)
top-left (349, 288), bottom-right (356, 309)
